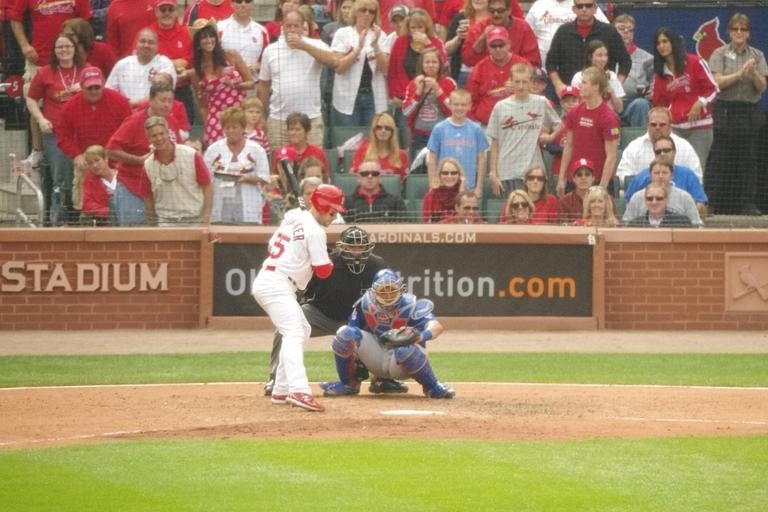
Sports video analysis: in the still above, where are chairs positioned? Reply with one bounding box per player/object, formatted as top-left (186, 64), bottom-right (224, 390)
top-left (0, 0), bottom-right (768, 227)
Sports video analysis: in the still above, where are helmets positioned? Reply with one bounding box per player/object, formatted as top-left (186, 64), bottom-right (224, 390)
top-left (309, 182), bottom-right (348, 216)
top-left (372, 269), bottom-right (406, 310)
top-left (334, 224), bottom-right (375, 276)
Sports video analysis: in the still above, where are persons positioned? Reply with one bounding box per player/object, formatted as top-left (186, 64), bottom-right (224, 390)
top-left (262, 226), bottom-right (410, 399)
top-left (245, 184), bottom-right (352, 412)
top-left (318, 266), bottom-right (456, 405)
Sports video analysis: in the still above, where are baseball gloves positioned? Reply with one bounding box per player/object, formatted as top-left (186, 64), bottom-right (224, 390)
top-left (380, 326), bottom-right (421, 350)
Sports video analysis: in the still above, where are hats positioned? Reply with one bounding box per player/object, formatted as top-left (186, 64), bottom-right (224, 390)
top-left (187, 15), bottom-right (219, 42)
top-left (568, 157), bottom-right (595, 176)
top-left (530, 64), bottom-right (549, 82)
top-left (485, 25), bottom-right (510, 46)
top-left (154, 0), bottom-right (178, 7)
top-left (386, 4), bottom-right (410, 22)
top-left (78, 65), bottom-right (105, 89)
top-left (561, 84), bottom-right (581, 100)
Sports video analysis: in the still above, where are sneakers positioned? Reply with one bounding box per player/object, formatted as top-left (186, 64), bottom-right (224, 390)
top-left (19, 149), bottom-right (45, 168)
top-left (367, 376), bottom-right (410, 396)
top-left (264, 384), bottom-right (289, 403)
top-left (422, 381), bottom-right (456, 398)
top-left (323, 381), bottom-right (360, 397)
top-left (284, 392), bottom-right (325, 413)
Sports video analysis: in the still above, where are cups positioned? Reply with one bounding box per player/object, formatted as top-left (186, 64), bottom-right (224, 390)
top-left (458, 18), bottom-right (469, 36)
top-left (413, 32), bottom-right (423, 53)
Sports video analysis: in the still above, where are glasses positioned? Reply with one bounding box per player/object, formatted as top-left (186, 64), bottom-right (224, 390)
top-left (511, 201), bottom-right (529, 209)
top-left (359, 170), bottom-right (380, 178)
top-left (527, 174), bottom-right (546, 182)
top-left (375, 124), bottom-right (392, 131)
top-left (488, 7), bottom-right (511, 13)
top-left (730, 27), bottom-right (750, 33)
top-left (231, 0), bottom-right (255, 4)
top-left (358, 7), bottom-right (377, 17)
top-left (649, 122), bottom-right (675, 156)
top-left (462, 206), bottom-right (480, 210)
top-left (576, 2), bottom-right (593, 9)
top-left (647, 196), bottom-right (666, 201)
top-left (159, 6), bottom-right (177, 13)
top-left (440, 170), bottom-right (459, 176)
top-left (575, 171), bottom-right (591, 177)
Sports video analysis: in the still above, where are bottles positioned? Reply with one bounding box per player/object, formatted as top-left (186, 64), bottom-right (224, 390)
top-left (334, 146), bottom-right (346, 173)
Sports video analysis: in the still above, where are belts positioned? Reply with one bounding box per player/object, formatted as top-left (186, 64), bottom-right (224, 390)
top-left (265, 265), bottom-right (298, 287)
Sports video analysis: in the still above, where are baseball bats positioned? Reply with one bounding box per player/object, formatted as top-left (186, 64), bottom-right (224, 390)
top-left (280, 157), bottom-right (307, 211)
